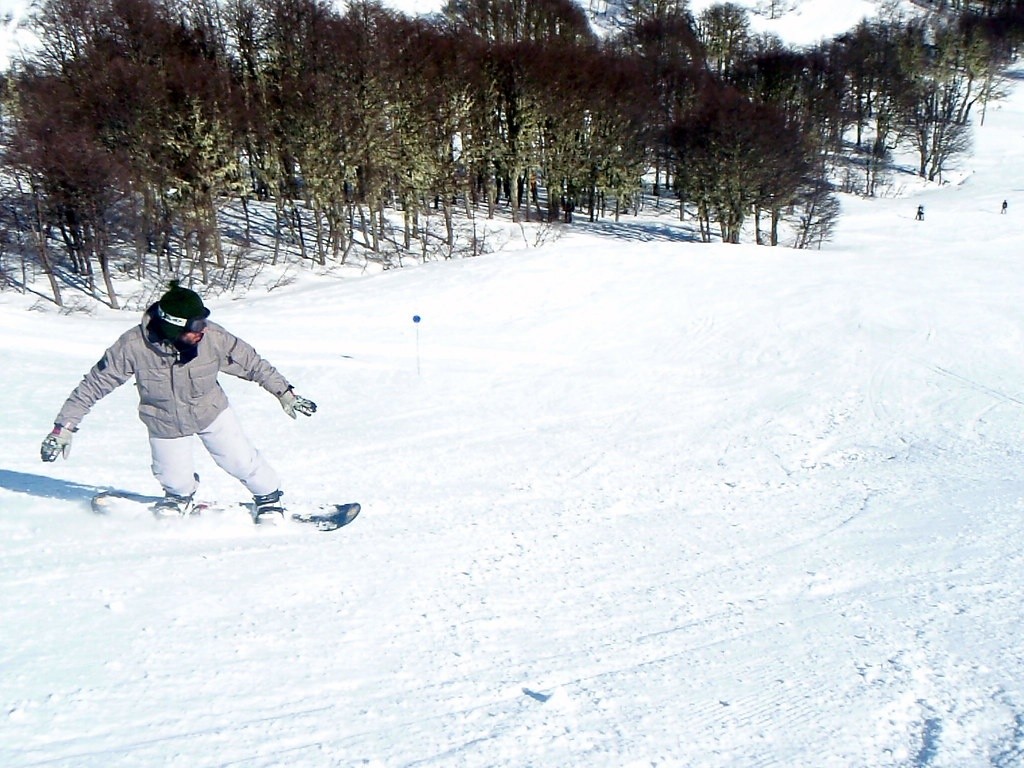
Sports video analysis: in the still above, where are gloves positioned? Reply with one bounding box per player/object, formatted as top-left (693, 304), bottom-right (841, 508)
top-left (41, 425), bottom-right (72, 462)
top-left (279, 389), bottom-right (317, 419)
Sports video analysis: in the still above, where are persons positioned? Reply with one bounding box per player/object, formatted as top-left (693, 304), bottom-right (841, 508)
top-left (1001, 200), bottom-right (1007, 214)
top-left (917, 204), bottom-right (924, 220)
top-left (565, 197), bottom-right (575, 222)
top-left (40, 280), bottom-right (316, 524)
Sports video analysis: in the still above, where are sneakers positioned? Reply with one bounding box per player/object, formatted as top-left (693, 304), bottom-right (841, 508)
top-left (252, 489), bottom-right (284, 525)
top-left (154, 473), bottom-right (199, 521)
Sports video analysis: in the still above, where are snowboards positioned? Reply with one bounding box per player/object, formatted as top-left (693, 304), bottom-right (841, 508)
top-left (89, 489), bottom-right (361, 532)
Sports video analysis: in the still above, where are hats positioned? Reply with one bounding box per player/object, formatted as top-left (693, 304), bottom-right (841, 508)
top-left (158, 279), bottom-right (211, 340)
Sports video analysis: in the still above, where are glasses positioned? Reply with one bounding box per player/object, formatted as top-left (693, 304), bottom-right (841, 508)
top-left (185, 318), bottom-right (207, 332)
